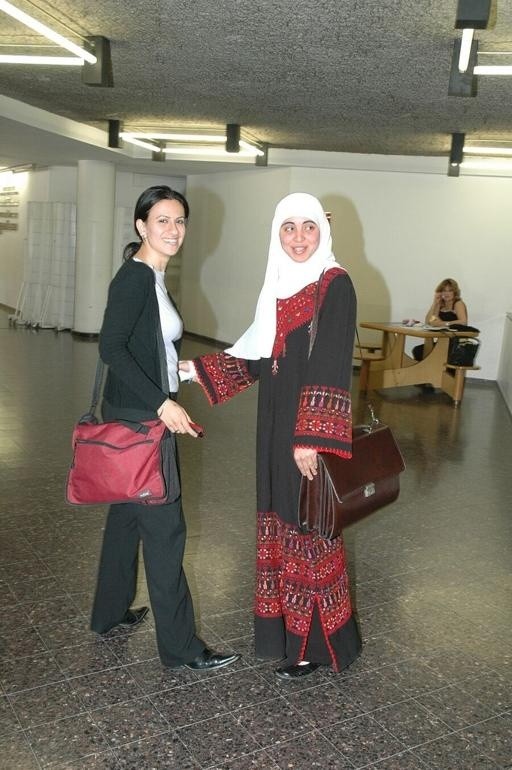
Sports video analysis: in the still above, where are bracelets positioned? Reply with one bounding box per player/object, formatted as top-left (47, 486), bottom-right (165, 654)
top-left (446, 321), bottom-right (451, 326)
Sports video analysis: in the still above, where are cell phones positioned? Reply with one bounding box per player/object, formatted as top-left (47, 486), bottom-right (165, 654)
top-left (191, 421), bottom-right (206, 438)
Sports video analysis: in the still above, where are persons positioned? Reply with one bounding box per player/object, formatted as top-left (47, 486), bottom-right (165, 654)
top-left (179, 191), bottom-right (363, 681)
top-left (90, 186), bottom-right (241, 670)
top-left (412, 278), bottom-right (468, 394)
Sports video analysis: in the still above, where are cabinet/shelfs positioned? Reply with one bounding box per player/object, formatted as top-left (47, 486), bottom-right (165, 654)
top-left (8, 201), bottom-right (76, 333)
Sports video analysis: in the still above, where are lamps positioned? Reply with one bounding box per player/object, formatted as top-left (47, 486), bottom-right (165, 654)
top-left (445, 132), bottom-right (512, 178)
top-left (446, 0), bottom-right (512, 99)
top-left (0, 0), bottom-right (114, 88)
top-left (108, 119), bottom-right (268, 167)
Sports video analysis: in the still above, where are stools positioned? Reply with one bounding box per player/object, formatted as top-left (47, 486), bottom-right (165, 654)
top-left (353, 351), bottom-right (386, 400)
top-left (355, 341), bottom-right (383, 354)
top-left (443, 363), bottom-right (481, 404)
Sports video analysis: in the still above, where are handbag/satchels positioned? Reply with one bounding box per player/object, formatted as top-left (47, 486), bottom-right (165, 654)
top-left (448, 335), bottom-right (480, 367)
top-left (296, 419), bottom-right (407, 542)
top-left (65, 410), bottom-right (184, 509)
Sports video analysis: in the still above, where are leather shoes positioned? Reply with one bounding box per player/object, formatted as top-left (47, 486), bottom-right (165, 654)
top-left (253, 651), bottom-right (290, 665)
top-left (273, 658), bottom-right (323, 679)
top-left (99, 606), bottom-right (151, 637)
top-left (164, 646), bottom-right (244, 672)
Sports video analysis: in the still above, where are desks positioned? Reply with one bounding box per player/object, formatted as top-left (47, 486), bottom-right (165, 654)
top-left (359, 322), bottom-right (479, 404)
